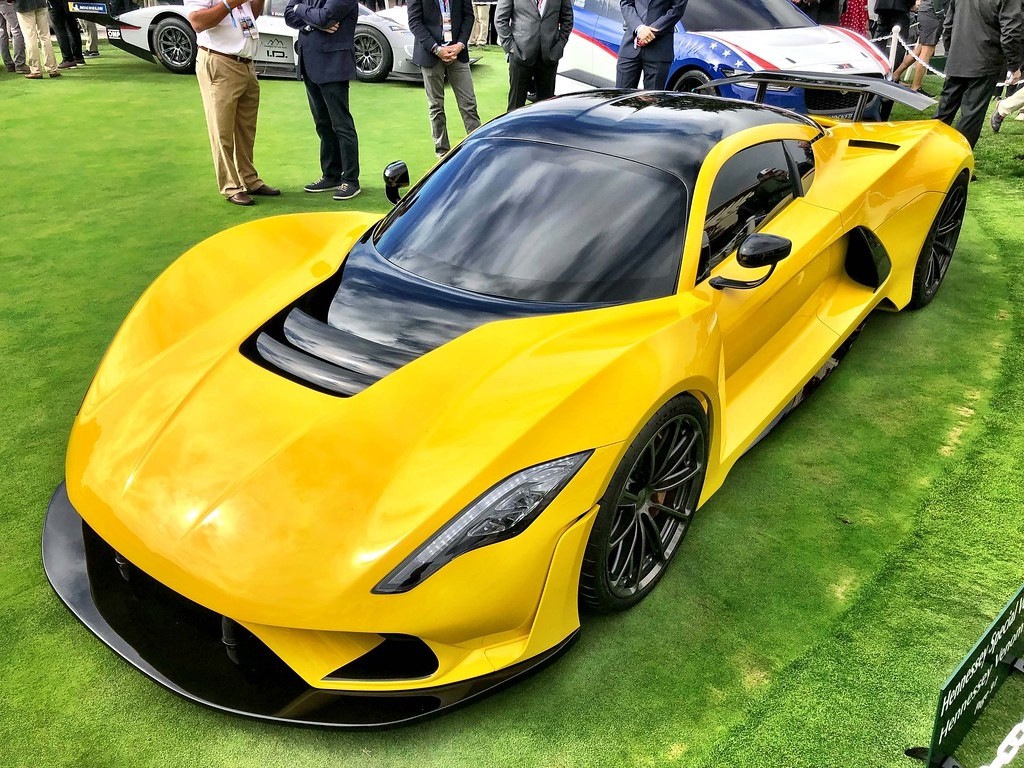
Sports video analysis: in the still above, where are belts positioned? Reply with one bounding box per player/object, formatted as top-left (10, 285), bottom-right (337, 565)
top-left (199, 46), bottom-right (252, 64)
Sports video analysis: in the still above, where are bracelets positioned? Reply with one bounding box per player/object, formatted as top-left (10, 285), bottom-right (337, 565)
top-left (223, 0), bottom-right (231, 12)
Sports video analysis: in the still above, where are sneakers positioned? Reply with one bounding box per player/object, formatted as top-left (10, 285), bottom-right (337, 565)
top-left (304, 176), bottom-right (342, 193)
top-left (332, 181), bottom-right (361, 200)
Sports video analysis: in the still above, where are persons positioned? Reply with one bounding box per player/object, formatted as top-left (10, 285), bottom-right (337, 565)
top-left (0, 0), bottom-right (31, 74)
top-left (46, 0), bottom-right (86, 70)
top-left (77, 17), bottom-right (100, 58)
top-left (12, 0), bottom-right (61, 79)
top-left (934, 0), bottom-right (1024, 182)
top-left (615, 0), bottom-right (688, 90)
top-left (407, 0), bottom-right (481, 159)
top-left (790, 0), bottom-right (1024, 133)
top-left (284, 0), bottom-right (361, 199)
top-left (179, 0), bottom-right (281, 205)
top-left (494, 0), bottom-right (574, 113)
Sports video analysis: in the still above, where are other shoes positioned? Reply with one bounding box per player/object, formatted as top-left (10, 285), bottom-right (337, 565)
top-left (74, 57), bottom-right (86, 66)
top-left (990, 99), bottom-right (1005, 133)
top-left (82, 50), bottom-right (93, 58)
top-left (92, 51), bottom-right (99, 56)
top-left (16, 64), bottom-right (30, 74)
top-left (890, 73), bottom-right (902, 86)
top-left (6, 64), bottom-right (15, 72)
top-left (915, 87), bottom-right (938, 99)
top-left (49, 71), bottom-right (60, 77)
top-left (57, 59), bottom-right (77, 69)
top-left (24, 73), bottom-right (43, 79)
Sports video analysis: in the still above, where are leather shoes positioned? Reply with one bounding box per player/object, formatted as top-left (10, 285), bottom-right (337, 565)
top-left (245, 185), bottom-right (281, 197)
top-left (226, 192), bottom-right (254, 206)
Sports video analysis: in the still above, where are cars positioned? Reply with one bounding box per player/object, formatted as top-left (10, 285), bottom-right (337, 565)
top-left (67, 0), bottom-right (485, 83)
top-left (505, 0), bottom-right (896, 122)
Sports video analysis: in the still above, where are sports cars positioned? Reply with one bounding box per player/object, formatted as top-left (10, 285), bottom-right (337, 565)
top-left (42, 68), bottom-right (977, 730)
